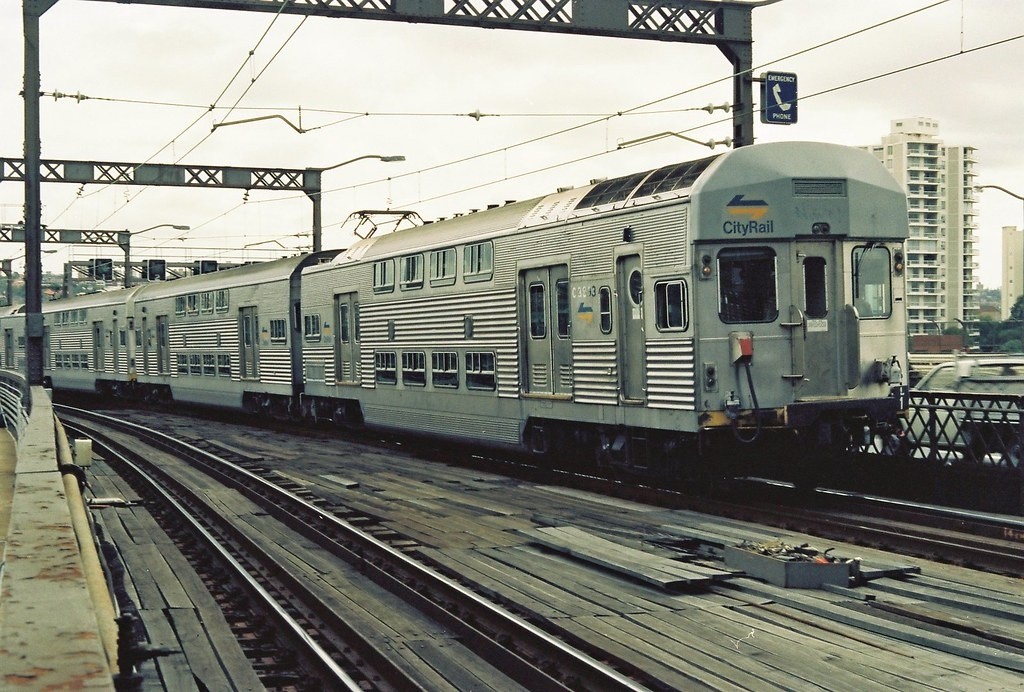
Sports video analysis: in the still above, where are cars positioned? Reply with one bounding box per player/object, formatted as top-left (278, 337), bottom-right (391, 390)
top-left (873, 358), bottom-right (1024, 468)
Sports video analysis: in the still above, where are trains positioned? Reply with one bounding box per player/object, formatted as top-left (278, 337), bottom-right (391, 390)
top-left (0, 142), bottom-right (912, 494)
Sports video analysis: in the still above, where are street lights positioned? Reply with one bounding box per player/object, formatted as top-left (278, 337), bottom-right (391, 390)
top-left (125, 224), bottom-right (191, 288)
top-left (312, 155), bottom-right (406, 252)
top-left (7, 249), bottom-right (57, 306)
